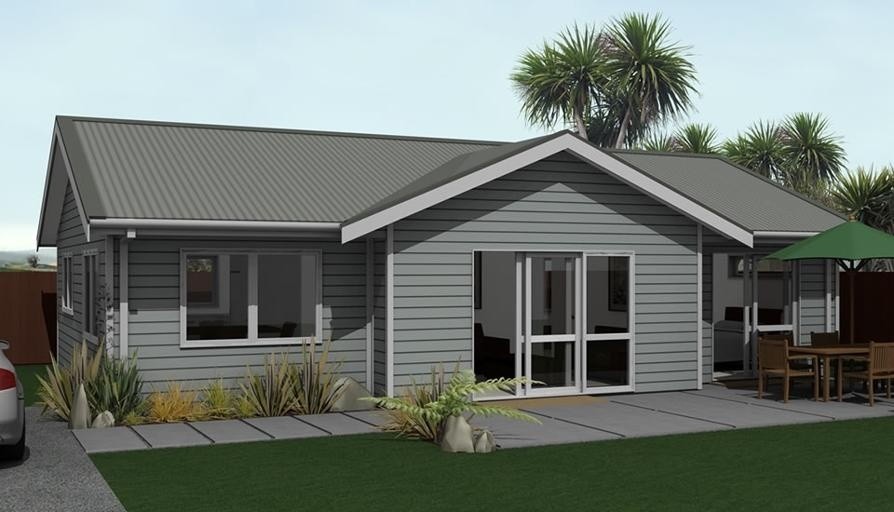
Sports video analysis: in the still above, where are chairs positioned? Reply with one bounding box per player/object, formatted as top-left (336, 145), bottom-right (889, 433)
top-left (756, 331), bottom-right (894, 407)
top-left (473, 323), bottom-right (510, 375)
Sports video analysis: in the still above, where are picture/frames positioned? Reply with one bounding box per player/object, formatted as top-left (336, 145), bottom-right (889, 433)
top-left (607, 256), bottom-right (630, 312)
